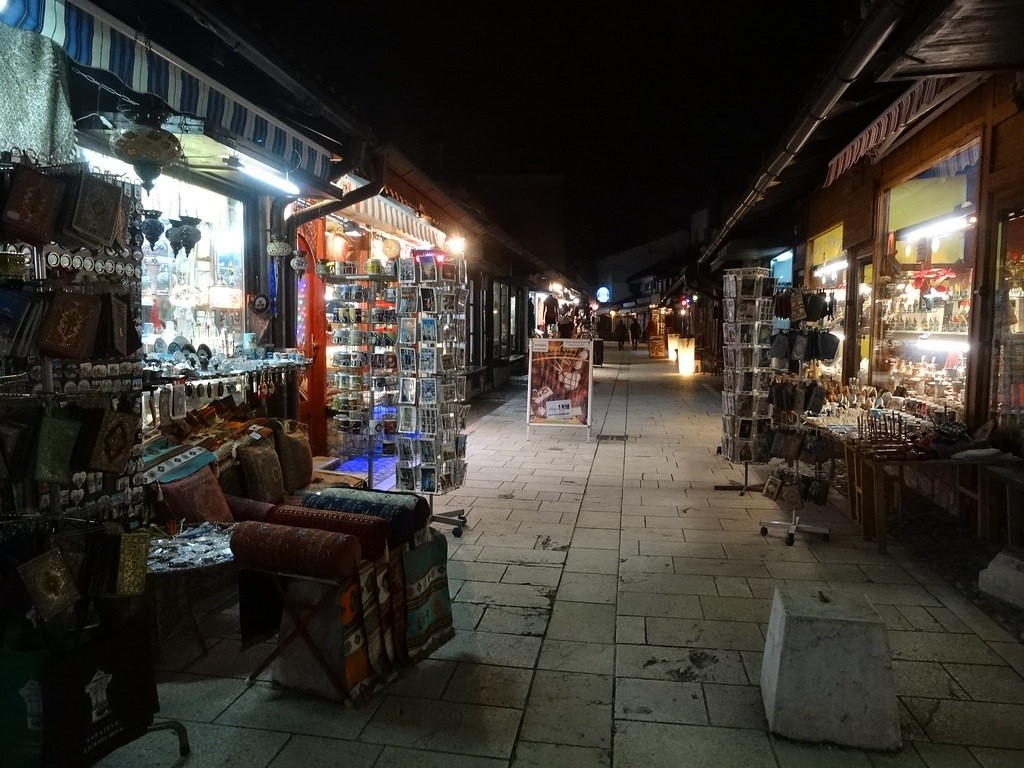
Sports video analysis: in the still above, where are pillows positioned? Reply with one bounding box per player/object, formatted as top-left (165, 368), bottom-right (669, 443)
top-left (149, 463), bottom-right (236, 529)
top-left (235, 433), bottom-right (287, 507)
top-left (278, 425), bottom-right (314, 495)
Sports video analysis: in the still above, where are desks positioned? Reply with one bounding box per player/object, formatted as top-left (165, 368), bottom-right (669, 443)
top-left (241, 536), bottom-right (412, 703)
top-left (844, 445), bottom-right (1024, 558)
top-left (141, 523), bottom-right (236, 658)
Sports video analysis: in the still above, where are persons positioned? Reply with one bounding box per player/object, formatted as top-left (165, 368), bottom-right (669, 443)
top-left (630, 319), bottom-right (642, 351)
top-left (615, 319), bottom-right (627, 351)
top-left (543, 295), bottom-right (558, 334)
top-left (557, 316), bottom-right (574, 339)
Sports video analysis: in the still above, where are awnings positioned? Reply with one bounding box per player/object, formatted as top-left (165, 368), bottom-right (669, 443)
top-left (343, 168), bottom-right (446, 245)
top-left (0, 0), bottom-right (331, 193)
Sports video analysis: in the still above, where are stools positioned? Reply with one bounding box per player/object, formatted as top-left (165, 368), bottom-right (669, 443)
top-left (694, 360), bottom-right (701, 372)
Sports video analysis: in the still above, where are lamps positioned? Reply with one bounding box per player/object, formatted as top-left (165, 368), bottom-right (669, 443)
top-left (342, 220), bottom-right (363, 237)
top-left (895, 204), bottom-right (976, 240)
top-left (667, 334), bottom-right (680, 362)
top-left (110, 104), bottom-right (329, 281)
top-left (677, 338), bottom-right (696, 380)
top-left (812, 254), bottom-right (848, 278)
